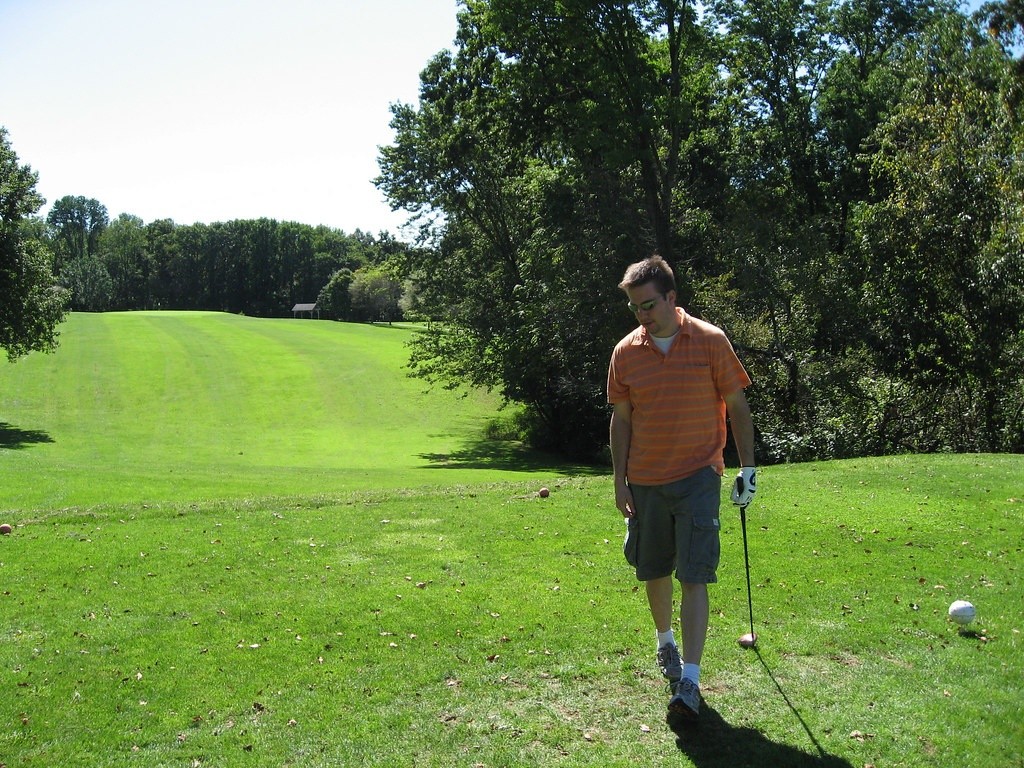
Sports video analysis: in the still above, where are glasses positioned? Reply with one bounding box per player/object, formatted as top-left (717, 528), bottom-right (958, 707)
top-left (628, 291), bottom-right (670, 313)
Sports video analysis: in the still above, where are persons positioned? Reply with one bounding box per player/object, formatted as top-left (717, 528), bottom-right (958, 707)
top-left (607, 255), bottom-right (757, 719)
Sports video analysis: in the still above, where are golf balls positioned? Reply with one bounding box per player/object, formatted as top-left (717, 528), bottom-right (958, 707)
top-left (539, 488), bottom-right (550, 497)
top-left (0, 524), bottom-right (12, 534)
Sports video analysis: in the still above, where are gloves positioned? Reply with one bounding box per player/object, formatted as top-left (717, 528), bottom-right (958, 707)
top-left (731, 465), bottom-right (756, 507)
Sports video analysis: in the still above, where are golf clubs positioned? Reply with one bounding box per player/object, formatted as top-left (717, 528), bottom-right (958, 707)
top-left (736, 477), bottom-right (758, 648)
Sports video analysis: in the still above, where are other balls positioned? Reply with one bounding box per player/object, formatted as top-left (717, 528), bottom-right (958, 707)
top-left (948, 600), bottom-right (975, 624)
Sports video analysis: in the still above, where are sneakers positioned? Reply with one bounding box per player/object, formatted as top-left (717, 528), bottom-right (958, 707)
top-left (664, 677), bottom-right (700, 716)
top-left (657, 640), bottom-right (682, 679)
